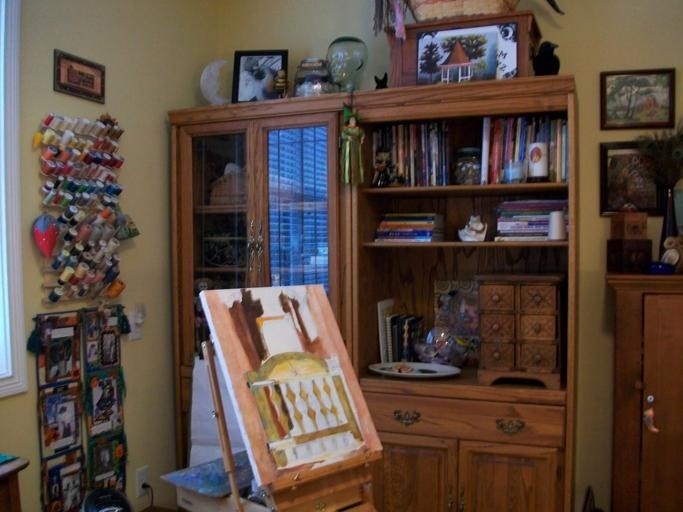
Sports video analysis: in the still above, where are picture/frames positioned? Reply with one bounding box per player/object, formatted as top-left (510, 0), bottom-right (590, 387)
top-left (231, 50), bottom-right (287, 104)
top-left (53, 47), bottom-right (106, 104)
top-left (598, 68), bottom-right (677, 129)
top-left (599, 143), bottom-right (659, 217)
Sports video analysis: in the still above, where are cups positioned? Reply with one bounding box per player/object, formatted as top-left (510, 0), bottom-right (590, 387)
top-left (527, 141), bottom-right (549, 177)
top-left (502, 159), bottom-right (524, 183)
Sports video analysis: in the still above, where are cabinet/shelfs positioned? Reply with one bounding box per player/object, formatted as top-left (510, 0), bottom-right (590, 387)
top-left (164, 88), bottom-right (350, 470)
top-left (349, 72), bottom-right (581, 391)
top-left (355, 383), bottom-right (573, 512)
top-left (472, 270), bottom-right (563, 392)
top-left (603, 273), bottom-right (682, 511)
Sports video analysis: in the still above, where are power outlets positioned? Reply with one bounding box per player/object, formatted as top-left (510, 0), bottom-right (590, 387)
top-left (137, 467), bottom-right (150, 497)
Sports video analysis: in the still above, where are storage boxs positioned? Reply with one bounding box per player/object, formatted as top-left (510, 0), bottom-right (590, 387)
top-left (607, 212), bottom-right (649, 240)
top-left (606, 238), bottom-right (651, 275)
top-left (382, 11), bottom-right (542, 85)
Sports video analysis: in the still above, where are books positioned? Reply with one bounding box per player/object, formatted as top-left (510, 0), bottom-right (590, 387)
top-left (375, 296), bottom-right (425, 363)
top-left (368, 118), bottom-right (570, 246)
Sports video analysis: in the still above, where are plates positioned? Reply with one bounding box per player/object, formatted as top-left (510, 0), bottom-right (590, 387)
top-left (368, 362), bottom-right (462, 379)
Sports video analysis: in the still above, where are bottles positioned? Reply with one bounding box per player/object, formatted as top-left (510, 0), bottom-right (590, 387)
top-left (548, 209), bottom-right (567, 241)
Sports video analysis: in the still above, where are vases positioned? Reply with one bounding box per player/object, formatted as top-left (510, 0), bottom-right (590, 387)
top-left (657, 188), bottom-right (682, 262)
